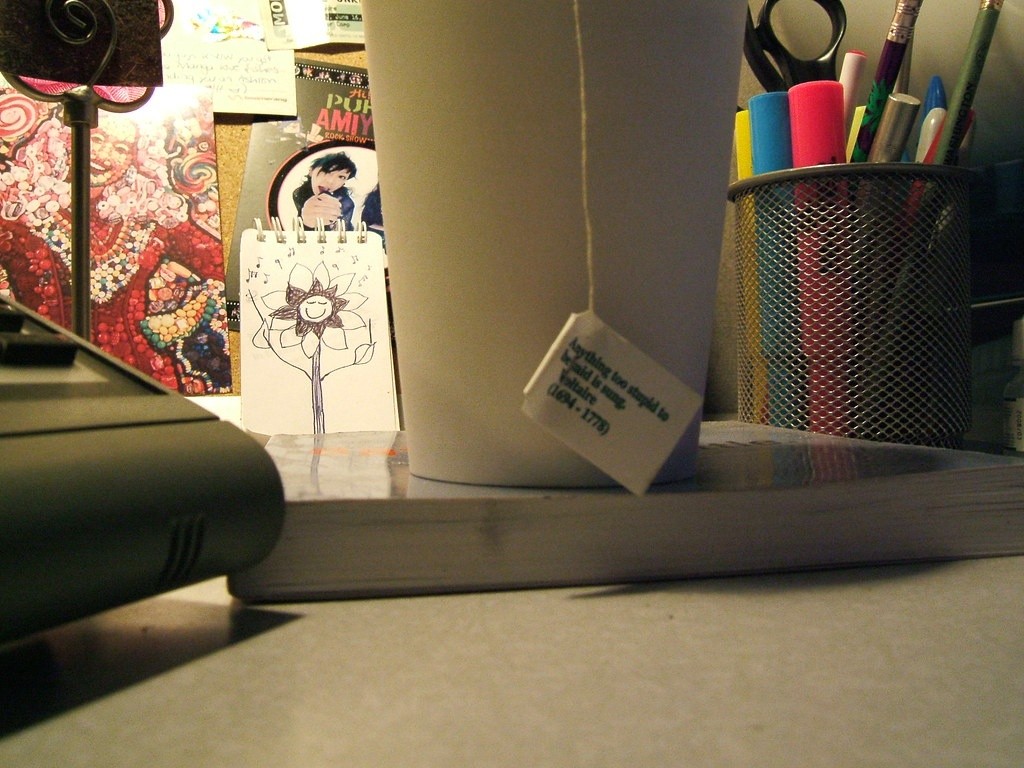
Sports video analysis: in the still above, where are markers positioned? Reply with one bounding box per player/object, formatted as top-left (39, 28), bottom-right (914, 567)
top-left (734, 79), bottom-right (849, 427)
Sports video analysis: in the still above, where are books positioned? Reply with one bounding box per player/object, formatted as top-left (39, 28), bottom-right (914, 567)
top-left (241, 215), bottom-right (401, 435)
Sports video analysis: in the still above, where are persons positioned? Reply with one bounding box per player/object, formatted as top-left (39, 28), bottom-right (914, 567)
top-left (360, 181), bottom-right (386, 255)
top-left (292, 152), bottom-right (357, 232)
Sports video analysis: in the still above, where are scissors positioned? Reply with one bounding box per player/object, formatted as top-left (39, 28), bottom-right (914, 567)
top-left (743, 2), bottom-right (848, 96)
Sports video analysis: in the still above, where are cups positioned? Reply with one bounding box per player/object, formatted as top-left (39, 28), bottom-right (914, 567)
top-left (360, 1), bottom-right (749, 488)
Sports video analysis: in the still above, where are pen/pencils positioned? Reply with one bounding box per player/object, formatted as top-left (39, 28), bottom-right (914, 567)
top-left (849, 1), bottom-right (922, 211)
top-left (856, 77), bottom-right (977, 220)
top-left (876, 1), bottom-right (1008, 387)
